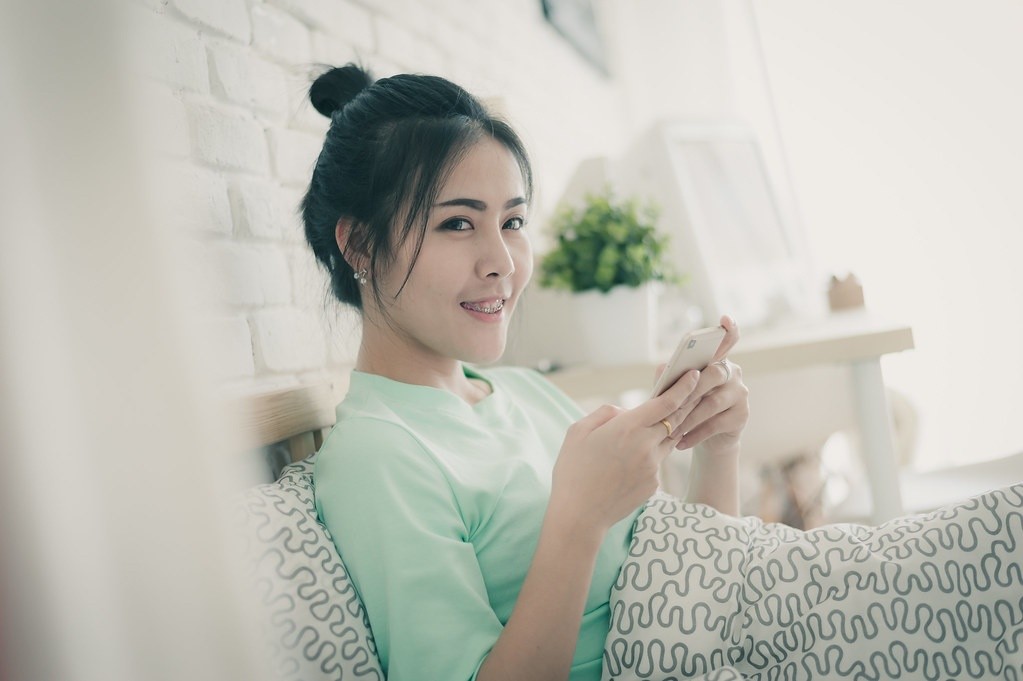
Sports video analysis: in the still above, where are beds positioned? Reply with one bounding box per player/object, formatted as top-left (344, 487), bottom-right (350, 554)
top-left (237, 376), bottom-right (1022, 681)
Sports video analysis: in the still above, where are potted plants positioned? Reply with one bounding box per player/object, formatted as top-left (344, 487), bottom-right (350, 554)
top-left (532, 193), bottom-right (673, 363)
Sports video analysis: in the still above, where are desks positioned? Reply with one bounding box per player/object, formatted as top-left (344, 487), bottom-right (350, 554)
top-left (554, 314), bottom-right (917, 533)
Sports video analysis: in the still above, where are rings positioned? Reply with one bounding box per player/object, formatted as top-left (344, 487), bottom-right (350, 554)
top-left (714, 360), bottom-right (730, 376)
top-left (660, 418), bottom-right (673, 437)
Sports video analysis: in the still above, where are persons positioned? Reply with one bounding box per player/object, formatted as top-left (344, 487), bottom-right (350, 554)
top-left (297, 64), bottom-right (749, 680)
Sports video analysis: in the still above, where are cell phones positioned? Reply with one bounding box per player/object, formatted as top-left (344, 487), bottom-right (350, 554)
top-left (651, 325), bottom-right (728, 401)
